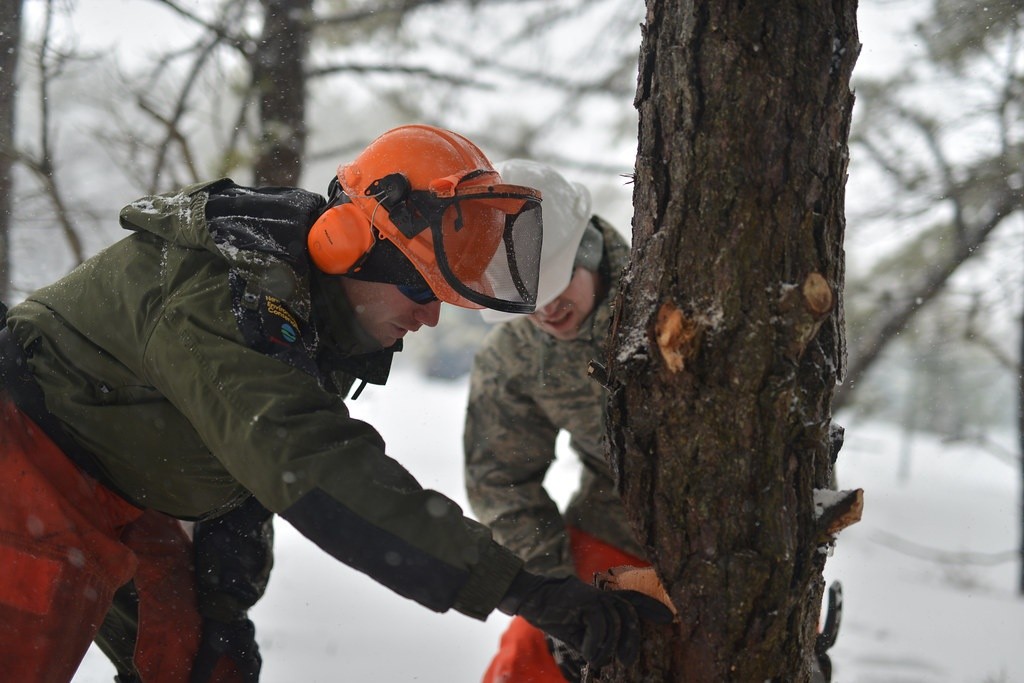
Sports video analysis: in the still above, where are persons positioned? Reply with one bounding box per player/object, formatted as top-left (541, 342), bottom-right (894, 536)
top-left (464, 159), bottom-right (652, 683)
top-left (1, 127), bottom-right (672, 682)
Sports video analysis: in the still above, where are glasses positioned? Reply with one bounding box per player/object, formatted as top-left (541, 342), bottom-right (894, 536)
top-left (376, 233), bottom-right (438, 306)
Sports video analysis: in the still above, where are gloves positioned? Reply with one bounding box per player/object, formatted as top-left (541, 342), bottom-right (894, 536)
top-left (514, 576), bottom-right (674, 668)
top-left (543, 632), bottom-right (587, 683)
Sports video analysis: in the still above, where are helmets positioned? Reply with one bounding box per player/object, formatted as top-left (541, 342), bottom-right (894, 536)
top-left (308, 124), bottom-right (508, 314)
top-left (478, 158), bottom-right (594, 324)
